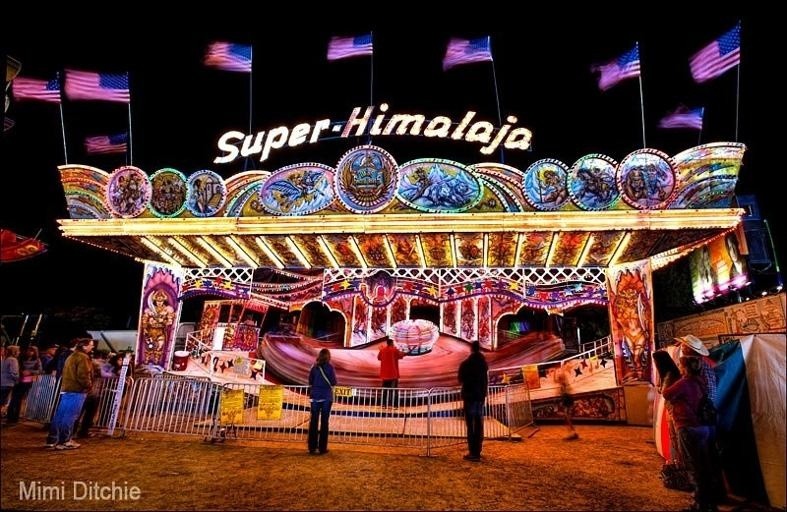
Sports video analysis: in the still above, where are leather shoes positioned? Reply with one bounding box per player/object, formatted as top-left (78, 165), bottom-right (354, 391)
top-left (463, 453), bottom-right (481, 461)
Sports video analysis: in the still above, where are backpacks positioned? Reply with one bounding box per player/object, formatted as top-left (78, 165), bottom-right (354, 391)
top-left (680, 375), bottom-right (717, 426)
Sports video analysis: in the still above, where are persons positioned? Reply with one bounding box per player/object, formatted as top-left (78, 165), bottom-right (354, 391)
top-left (725, 233), bottom-right (749, 284)
top-left (553, 370), bottom-right (578, 440)
top-left (661, 355), bottom-right (719, 512)
top-left (703, 246), bottom-right (717, 289)
top-left (673, 334), bottom-right (728, 503)
top-left (309, 349), bottom-right (337, 455)
top-left (0, 337), bottom-right (124, 450)
top-left (457, 342), bottom-right (489, 462)
top-left (140, 292), bottom-right (172, 365)
top-left (377, 339), bottom-right (409, 410)
top-left (653, 351), bottom-right (685, 491)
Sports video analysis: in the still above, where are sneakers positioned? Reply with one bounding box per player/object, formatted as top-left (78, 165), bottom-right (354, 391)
top-left (55, 439), bottom-right (81, 450)
top-left (45, 442), bottom-right (59, 449)
top-left (682, 500), bottom-right (719, 512)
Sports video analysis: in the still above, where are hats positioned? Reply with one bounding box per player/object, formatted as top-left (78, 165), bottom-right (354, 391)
top-left (673, 334), bottom-right (710, 357)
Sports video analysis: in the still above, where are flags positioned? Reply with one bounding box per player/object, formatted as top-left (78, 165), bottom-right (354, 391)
top-left (11, 77), bottom-right (61, 103)
top-left (657, 105), bottom-right (704, 131)
top-left (590, 46), bottom-right (641, 92)
top-left (63, 69), bottom-right (130, 103)
top-left (688, 23), bottom-right (741, 83)
top-left (202, 41), bottom-right (252, 72)
top-left (442, 35), bottom-right (493, 72)
top-left (84, 134), bottom-right (127, 154)
top-left (326, 32), bottom-right (373, 62)
